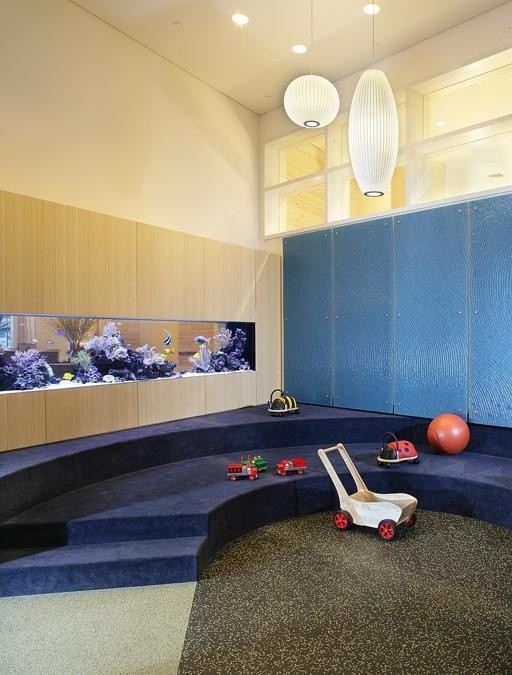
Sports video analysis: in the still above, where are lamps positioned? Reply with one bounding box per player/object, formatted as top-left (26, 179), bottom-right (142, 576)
top-left (284, 1), bottom-right (340, 129)
top-left (348, 0), bottom-right (399, 197)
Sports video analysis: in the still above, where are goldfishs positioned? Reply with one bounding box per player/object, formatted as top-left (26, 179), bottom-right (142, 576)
top-left (63, 372), bottom-right (75, 380)
